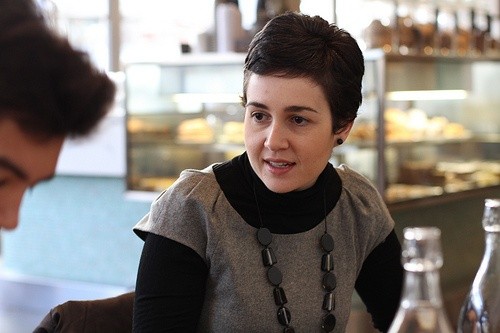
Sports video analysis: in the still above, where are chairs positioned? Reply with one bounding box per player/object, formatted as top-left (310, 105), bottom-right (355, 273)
top-left (34, 292), bottom-right (134, 333)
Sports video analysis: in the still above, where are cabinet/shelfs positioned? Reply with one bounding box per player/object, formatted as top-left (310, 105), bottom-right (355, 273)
top-left (126, 49), bottom-right (500, 204)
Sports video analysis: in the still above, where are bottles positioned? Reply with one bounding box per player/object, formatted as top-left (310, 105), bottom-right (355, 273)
top-left (457, 200), bottom-right (500, 333)
top-left (387, 226), bottom-right (455, 333)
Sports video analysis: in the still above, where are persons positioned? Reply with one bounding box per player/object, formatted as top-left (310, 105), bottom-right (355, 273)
top-left (131, 13), bottom-right (410, 333)
top-left (0, 0), bottom-right (120, 237)
top-left (250, 168), bottom-right (337, 333)
